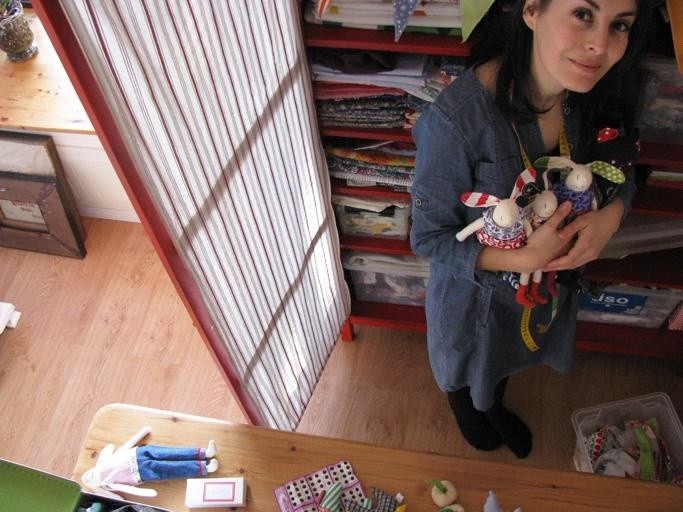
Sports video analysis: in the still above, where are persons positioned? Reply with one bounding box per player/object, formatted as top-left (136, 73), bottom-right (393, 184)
top-left (405, 1), bottom-right (655, 460)
top-left (81, 428), bottom-right (220, 499)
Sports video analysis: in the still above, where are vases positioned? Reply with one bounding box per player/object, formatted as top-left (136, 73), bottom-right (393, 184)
top-left (0, 1), bottom-right (38, 66)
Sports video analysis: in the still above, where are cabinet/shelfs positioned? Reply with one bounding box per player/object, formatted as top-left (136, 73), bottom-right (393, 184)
top-left (28, 2), bottom-right (683, 430)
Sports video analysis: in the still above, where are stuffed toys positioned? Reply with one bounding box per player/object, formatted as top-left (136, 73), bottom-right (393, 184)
top-left (459, 155), bottom-right (627, 308)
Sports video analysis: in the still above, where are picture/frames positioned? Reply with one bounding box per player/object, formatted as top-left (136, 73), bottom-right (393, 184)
top-left (0, 130), bottom-right (90, 244)
top-left (0, 169), bottom-right (89, 261)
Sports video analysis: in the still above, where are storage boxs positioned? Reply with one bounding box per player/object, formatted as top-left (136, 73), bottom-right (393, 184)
top-left (628, 54), bottom-right (683, 151)
top-left (558, 387), bottom-right (683, 487)
top-left (334, 198), bottom-right (411, 240)
top-left (340, 251), bottom-right (431, 309)
top-left (576, 283), bottom-right (682, 334)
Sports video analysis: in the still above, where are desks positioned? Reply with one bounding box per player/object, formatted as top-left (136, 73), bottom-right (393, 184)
top-left (70, 402), bottom-right (682, 511)
top-left (0, 7), bottom-right (147, 223)
